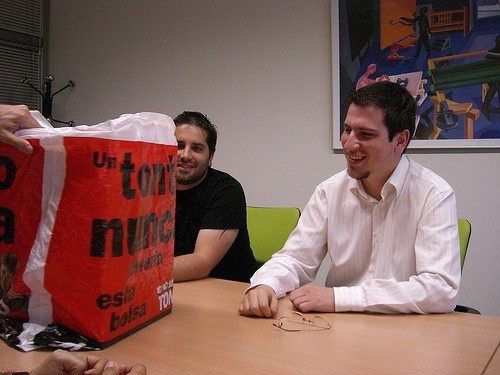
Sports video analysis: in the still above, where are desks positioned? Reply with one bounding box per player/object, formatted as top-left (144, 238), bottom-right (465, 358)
top-left (0, 275), bottom-right (500, 375)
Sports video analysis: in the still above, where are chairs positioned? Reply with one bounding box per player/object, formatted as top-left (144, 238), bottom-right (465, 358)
top-left (458, 218), bottom-right (483, 313)
top-left (246, 206), bottom-right (301, 264)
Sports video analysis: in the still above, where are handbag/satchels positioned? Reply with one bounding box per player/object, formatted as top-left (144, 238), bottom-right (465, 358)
top-left (1, 108), bottom-right (178, 352)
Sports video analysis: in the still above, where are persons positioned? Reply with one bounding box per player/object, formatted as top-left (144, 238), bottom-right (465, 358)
top-left (237, 81), bottom-right (461, 317)
top-left (173, 110), bottom-right (257, 284)
top-left (0, 104), bottom-right (42, 153)
top-left (0, 350), bottom-right (148, 375)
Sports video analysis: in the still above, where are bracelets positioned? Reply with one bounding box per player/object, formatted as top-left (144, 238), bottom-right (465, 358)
top-left (12, 371), bottom-right (29, 375)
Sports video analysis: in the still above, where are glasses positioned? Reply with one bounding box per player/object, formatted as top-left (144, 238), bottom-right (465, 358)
top-left (272, 310), bottom-right (331, 332)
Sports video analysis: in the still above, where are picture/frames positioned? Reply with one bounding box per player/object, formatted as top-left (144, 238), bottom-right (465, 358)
top-left (330, 0), bottom-right (500, 149)
